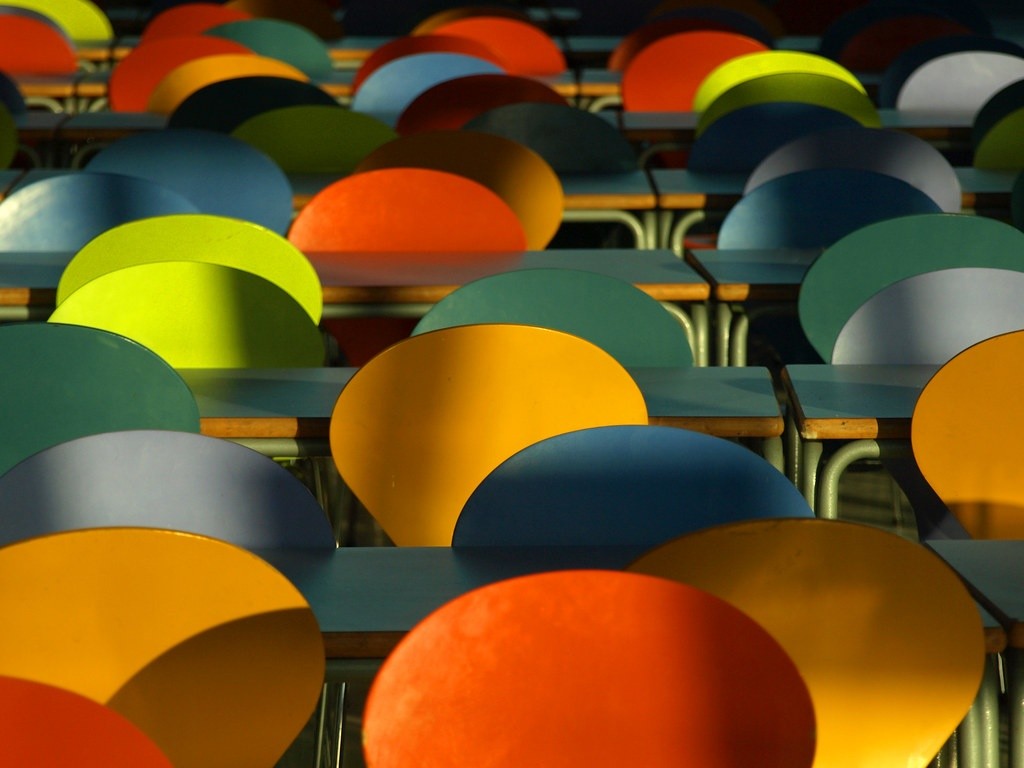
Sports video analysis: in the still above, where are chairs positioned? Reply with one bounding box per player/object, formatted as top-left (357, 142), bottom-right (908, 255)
top-left (0, 0), bottom-right (1024, 768)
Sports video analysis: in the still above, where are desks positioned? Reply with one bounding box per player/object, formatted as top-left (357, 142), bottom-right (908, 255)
top-left (0, 167), bottom-right (657, 250)
top-left (649, 170), bottom-right (1023, 261)
top-left (276, 544), bottom-right (1009, 767)
top-left (689, 247), bottom-right (822, 368)
top-left (782, 363), bottom-right (940, 520)
top-left (63, 113), bottom-right (622, 166)
top-left (9, 112), bottom-right (68, 169)
top-left (13, 39), bottom-right (903, 109)
top-left (0, 170), bottom-right (26, 198)
top-left (0, 251), bottom-right (709, 368)
top-left (621, 108), bottom-right (977, 173)
top-left (926, 537), bottom-right (1024, 768)
top-left (173, 367), bottom-right (783, 478)
